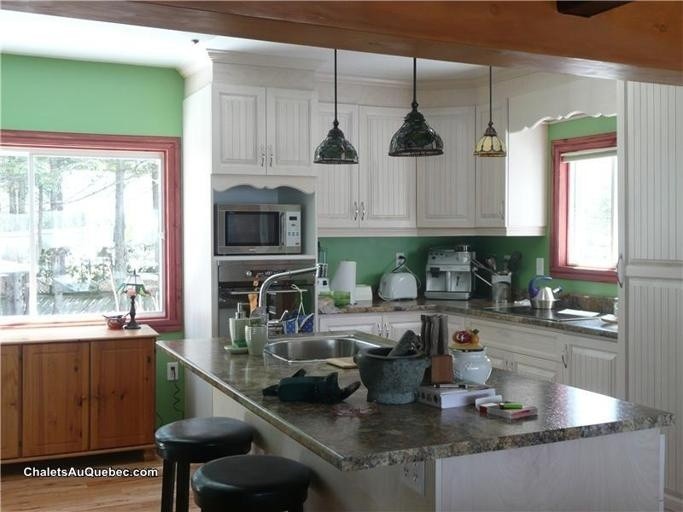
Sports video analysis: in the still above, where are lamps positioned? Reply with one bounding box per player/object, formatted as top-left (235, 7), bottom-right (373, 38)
top-left (472, 64), bottom-right (507, 158)
top-left (387, 57), bottom-right (444, 158)
top-left (313, 48), bottom-right (359, 164)
top-left (116, 269), bottom-right (151, 329)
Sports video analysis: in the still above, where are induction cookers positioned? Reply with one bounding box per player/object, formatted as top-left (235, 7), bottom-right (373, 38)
top-left (482, 303), bottom-right (594, 322)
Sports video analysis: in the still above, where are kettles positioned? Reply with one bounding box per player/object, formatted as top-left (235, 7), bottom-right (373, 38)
top-left (525, 272), bottom-right (563, 309)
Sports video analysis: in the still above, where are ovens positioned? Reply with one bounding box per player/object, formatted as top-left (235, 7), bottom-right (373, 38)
top-left (215, 259), bottom-right (315, 341)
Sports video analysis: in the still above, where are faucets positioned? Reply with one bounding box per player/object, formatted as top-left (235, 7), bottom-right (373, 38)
top-left (258, 263), bottom-right (328, 325)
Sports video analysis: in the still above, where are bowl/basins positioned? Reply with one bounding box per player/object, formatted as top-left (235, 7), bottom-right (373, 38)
top-left (318, 290), bottom-right (351, 306)
top-left (353, 344), bottom-right (430, 405)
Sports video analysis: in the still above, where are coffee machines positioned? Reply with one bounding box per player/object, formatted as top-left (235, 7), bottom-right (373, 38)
top-left (423, 243), bottom-right (474, 301)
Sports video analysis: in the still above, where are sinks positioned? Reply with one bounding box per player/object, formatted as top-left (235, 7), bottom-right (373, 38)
top-left (264, 334), bottom-right (396, 364)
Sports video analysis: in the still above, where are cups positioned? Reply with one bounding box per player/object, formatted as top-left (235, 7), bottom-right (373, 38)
top-left (612, 297), bottom-right (619, 317)
top-left (243, 324), bottom-right (267, 353)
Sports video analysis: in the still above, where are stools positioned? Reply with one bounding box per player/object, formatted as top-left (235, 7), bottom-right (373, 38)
top-left (190, 454), bottom-right (311, 512)
top-left (153, 414), bottom-right (254, 511)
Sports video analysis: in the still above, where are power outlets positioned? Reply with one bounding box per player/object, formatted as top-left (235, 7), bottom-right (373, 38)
top-left (394, 252), bottom-right (405, 269)
top-left (166, 361), bottom-right (178, 381)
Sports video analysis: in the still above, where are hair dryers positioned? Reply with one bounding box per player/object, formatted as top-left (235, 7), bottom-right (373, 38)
top-left (330, 261), bottom-right (357, 305)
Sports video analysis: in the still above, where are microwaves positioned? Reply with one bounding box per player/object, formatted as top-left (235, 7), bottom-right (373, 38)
top-left (212, 201), bottom-right (303, 254)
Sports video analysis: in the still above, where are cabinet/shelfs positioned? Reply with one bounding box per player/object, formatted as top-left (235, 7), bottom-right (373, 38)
top-left (182, 49), bottom-right (319, 176)
top-left (319, 311), bottom-right (437, 343)
top-left (565, 332), bottom-right (619, 395)
top-left (314, 75), bottom-right (416, 238)
top-left (464, 317), bottom-right (563, 382)
top-left (0, 323), bottom-right (158, 465)
top-left (616, 79), bottom-right (683, 512)
top-left (417, 69), bottom-right (549, 235)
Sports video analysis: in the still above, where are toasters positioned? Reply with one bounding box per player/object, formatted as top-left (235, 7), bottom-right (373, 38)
top-left (377, 273), bottom-right (417, 300)
